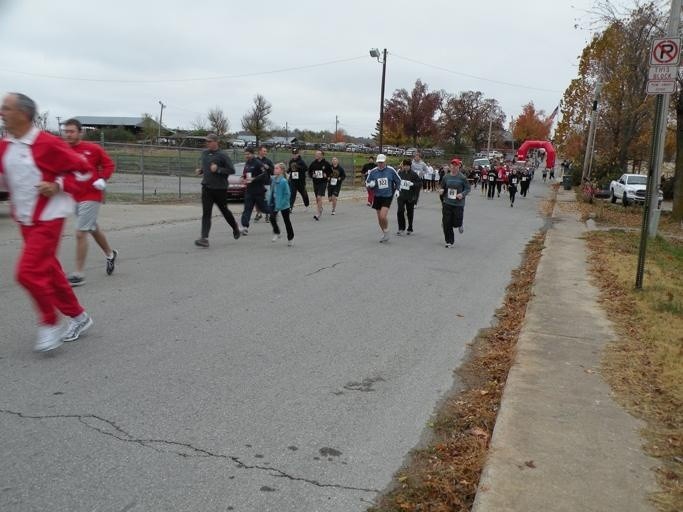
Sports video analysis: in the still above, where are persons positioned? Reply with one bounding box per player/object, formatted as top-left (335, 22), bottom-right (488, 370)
top-left (194, 132), bottom-right (241, 248)
top-left (361, 151), bottom-right (428, 243)
top-left (438, 159), bottom-right (471, 249)
top-left (422, 148), bottom-right (554, 207)
top-left (240, 145), bottom-right (346, 246)
top-left (0, 93), bottom-right (96, 355)
top-left (62, 118), bottom-right (119, 286)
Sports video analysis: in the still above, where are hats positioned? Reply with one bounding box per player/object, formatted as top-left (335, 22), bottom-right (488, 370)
top-left (204, 134), bottom-right (218, 142)
top-left (292, 148), bottom-right (298, 154)
top-left (376, 154), bottom-right (386, 163)
top-left (450, 158), bottom-right (460, 165)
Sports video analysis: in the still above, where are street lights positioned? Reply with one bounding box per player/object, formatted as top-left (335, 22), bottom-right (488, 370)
top-left (369, 47), bottom-right (388, 155)
top-left (159, 101), bottom-right (166, 145)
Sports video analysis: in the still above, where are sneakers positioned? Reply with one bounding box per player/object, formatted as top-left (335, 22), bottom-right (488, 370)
top-left (33, 251), bottom-right (117, 351)
top-left (195, 201), bottom-right (464, 248)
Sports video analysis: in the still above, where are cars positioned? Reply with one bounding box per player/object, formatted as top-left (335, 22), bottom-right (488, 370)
top-left (226, 161), bottom-right (247, 199)
top-left (472, 149), bottom-right (516, 180)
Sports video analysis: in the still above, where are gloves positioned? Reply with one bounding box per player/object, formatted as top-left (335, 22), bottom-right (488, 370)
top-left (93, 178), bottom-right (106, 190)
top-left (370, 180), bottom-right (414, 199)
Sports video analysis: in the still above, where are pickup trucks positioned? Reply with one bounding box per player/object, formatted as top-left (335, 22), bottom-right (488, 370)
top-left (610, 173), bottom-right (664, 208)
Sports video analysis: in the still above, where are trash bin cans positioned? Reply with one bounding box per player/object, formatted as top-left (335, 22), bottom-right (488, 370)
top-left (562, 175), bottom-right (572, 191)
